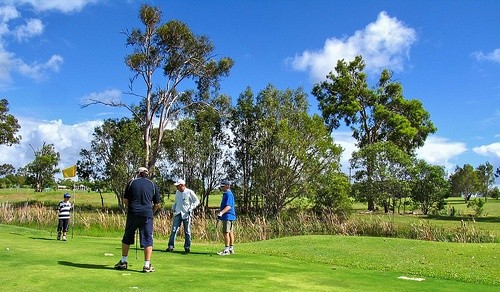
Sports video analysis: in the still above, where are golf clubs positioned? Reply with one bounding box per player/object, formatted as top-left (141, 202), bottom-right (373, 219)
top-left (210, 219), bottom-right (219, 256)
top-left (136, 228), bottom-right (138, 259)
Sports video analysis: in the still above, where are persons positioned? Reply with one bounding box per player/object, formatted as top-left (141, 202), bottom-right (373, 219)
top-left (216, 180), bottom-right (236, 255)
top-left (36, 183), bottom-right (39, 192)
top-left (165, 179), bottom-right (200, 254)
top-left (56, 193), bottom-right (73, 241)
top-left (114, 167), bottom-right (161, 272)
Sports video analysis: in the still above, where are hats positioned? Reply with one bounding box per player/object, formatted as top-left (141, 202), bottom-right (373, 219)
top-left (135, 166), bottom-right (149, 176)
top-left (174, 179), bottom-right (185, 185)
top-left (219, 181), bottom-right (230, 187)
top-left (64, 193), bottom-right (72, 198)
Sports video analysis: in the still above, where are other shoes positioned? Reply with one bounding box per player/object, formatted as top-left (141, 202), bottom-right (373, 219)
top-left (143, 264), bottom-right (155, 272)
top-left (216, 249), bottom-right (234, 256)
top-left (185, 248), bottom-right (190, 253)
top-left (166, 248), bottom-right (173, 252)
top-left (57, 233), bottom-right (61, 240)
top-left (114, 260), bottom-right (128, 270)
top-left (62, 236), bottom-right (67, 241)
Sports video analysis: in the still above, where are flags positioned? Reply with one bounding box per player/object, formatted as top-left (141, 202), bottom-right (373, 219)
top-left (62, 165), bottom-right (74, 178)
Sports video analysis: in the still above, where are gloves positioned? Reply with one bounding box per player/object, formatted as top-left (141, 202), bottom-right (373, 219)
top-left (217, 212), bottom-right (223, 219)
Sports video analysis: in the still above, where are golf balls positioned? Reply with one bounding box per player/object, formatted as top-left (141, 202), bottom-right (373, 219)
top-left (6, 248), bottom-right (10, 251)
